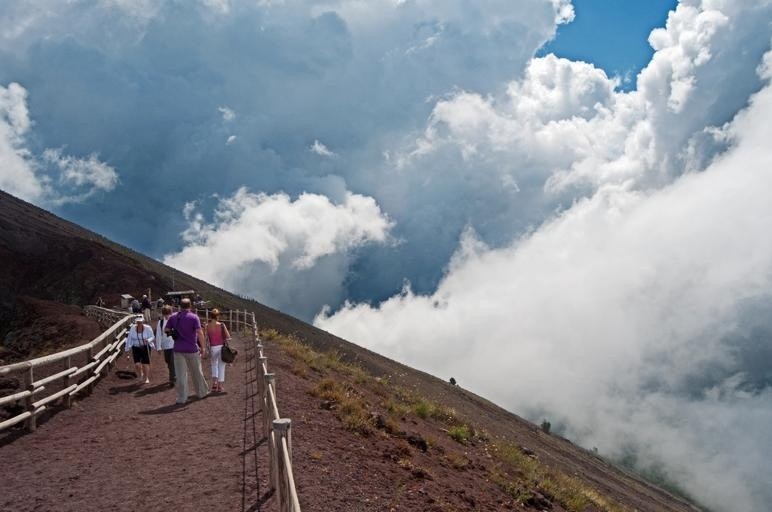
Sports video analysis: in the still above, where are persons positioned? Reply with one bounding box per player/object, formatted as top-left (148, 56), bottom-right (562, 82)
top-left (124, 294), bottom-right (231, 406)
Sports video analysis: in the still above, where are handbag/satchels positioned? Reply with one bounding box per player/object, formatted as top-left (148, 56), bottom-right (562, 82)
top-left (221, 346), bottom-right (238, 363)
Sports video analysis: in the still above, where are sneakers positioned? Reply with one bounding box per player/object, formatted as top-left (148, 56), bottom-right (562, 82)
top-left (212, 385), bottom-right (225, 393)
top-left (136, 377), bottom-right (150, 384)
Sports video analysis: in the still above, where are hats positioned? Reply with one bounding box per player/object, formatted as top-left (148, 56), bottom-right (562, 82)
top-left (134, 313), bottom-right (145, 322)
